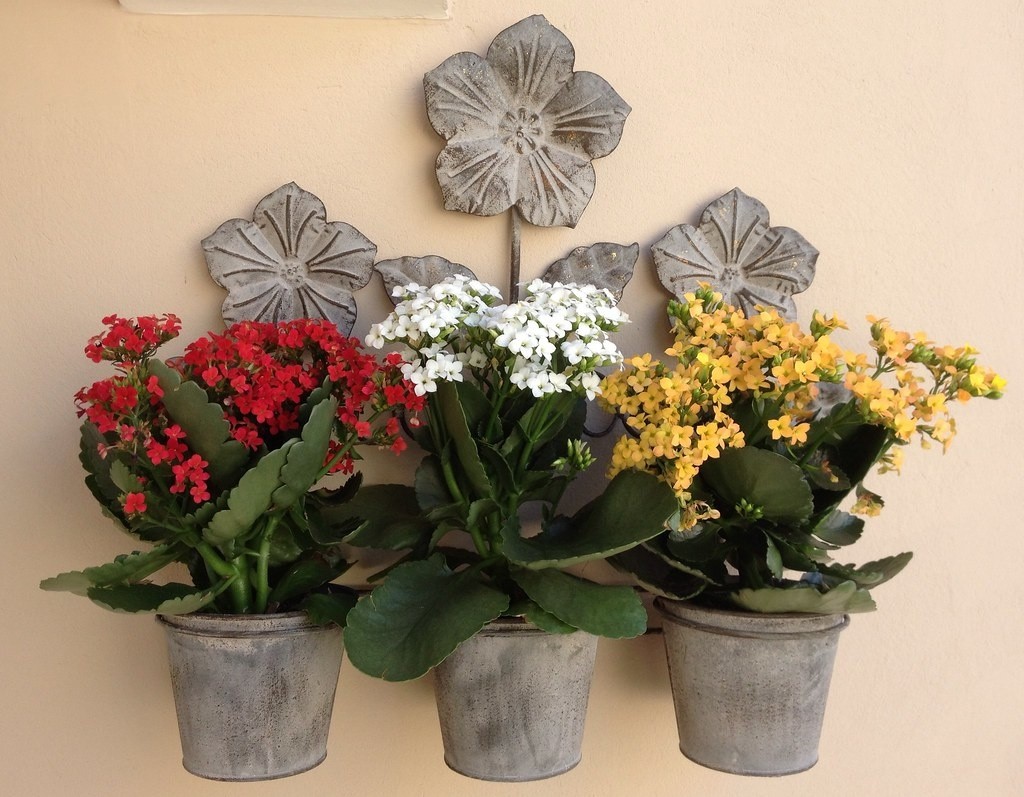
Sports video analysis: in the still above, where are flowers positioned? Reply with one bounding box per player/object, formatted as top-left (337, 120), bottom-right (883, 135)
top-left (39, 304), bottom-right (431, 636)
top-left (594, 280), bottom-right (1008, 614)
top-left (336, 269), bottom-right (681, 684)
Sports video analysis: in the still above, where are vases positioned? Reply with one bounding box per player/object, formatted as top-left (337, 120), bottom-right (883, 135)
top-left (430, 612), bottom-right (600, 784)
top-left (153, 583), bottom-right (361, 783)
top-left (652, 574), bottom-right (851, 779)
